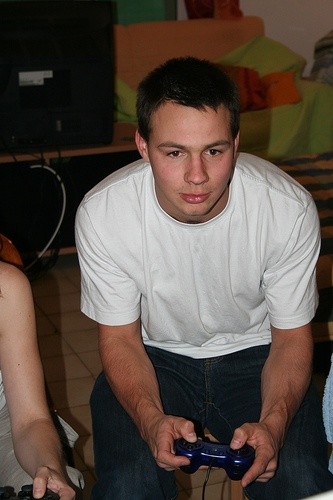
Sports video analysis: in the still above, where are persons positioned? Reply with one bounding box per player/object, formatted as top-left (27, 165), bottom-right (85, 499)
top-left (74, 56), bottom-right (333, 500)
top-left (0, 260), bottom-right (84, 500)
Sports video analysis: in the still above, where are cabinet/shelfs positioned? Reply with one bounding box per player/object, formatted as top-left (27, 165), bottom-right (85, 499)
top-left (0, 123), bottom-right (137, 258)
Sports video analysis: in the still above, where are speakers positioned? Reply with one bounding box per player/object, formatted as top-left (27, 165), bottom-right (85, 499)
top-left (0, 0), bottom-right (114, 154)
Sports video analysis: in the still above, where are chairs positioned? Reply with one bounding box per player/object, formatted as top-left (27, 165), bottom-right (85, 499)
top-left (0, 234), bottom-right (26, 275)
top-left (195, 253), bottom-right (332, 500)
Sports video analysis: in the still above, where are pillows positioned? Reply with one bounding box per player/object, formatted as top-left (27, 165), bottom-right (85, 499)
top-left (212, 62), bottom-right (264, 113)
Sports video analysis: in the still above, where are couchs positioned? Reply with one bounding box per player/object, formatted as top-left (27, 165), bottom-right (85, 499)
top-left (115, 15), bottom-right (265, 91)
